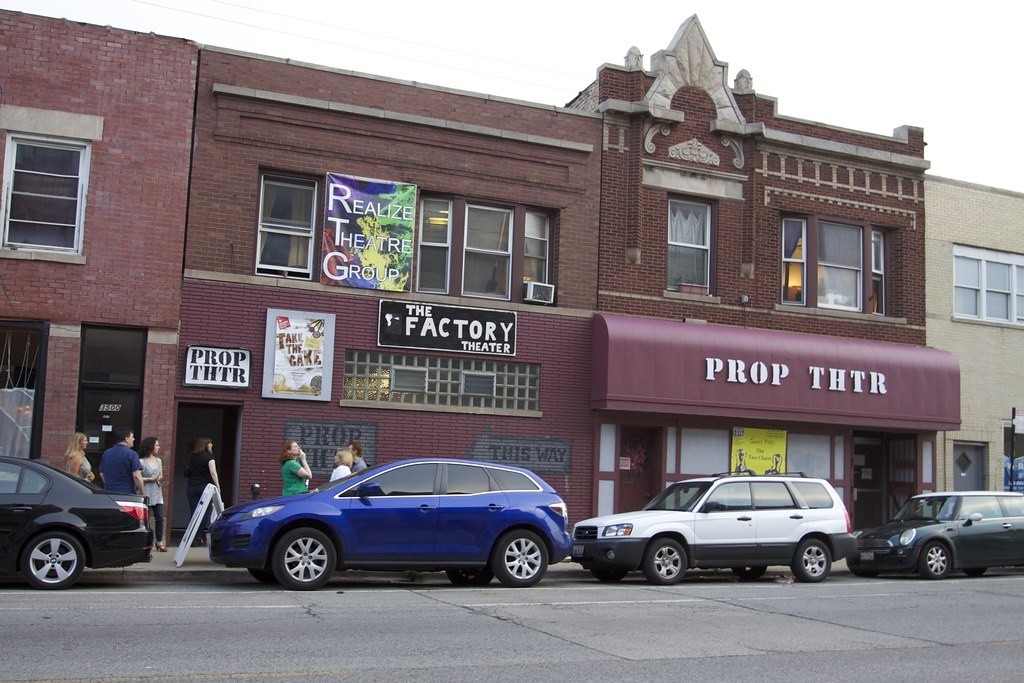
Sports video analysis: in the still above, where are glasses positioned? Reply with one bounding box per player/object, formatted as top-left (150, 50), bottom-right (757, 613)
top-left (290, 445), bottom-right (300, 448)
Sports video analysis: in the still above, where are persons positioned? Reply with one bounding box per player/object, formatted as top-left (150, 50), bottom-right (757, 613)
top-left (330, 442), bottom-right (367, 482)
top-left (184, 436), bottom-right (220, 546)
top-left (139, 437), bottom-right (168, 552)
top-left (64, 432), bottom-right (95, 483)
top-left (99, 427), bottom-right (147, 495)
top-left (280, 440), bottom-right (312, 496)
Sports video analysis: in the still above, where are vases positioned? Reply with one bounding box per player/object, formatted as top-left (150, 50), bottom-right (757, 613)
top-left (677, 283), bottom-right (708, 296)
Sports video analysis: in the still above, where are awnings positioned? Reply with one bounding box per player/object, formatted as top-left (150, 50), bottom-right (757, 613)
top-left (590, 309), bottom-right (962, 430)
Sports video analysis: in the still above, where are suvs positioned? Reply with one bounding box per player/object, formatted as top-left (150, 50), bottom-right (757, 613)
top-left (205, 457), bottom-right (574, 593)
top-left (570, 468), bottom-right (860, 586)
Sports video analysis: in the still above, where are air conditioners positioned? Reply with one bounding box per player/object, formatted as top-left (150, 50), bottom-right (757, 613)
top-left (523, 281), bottom-right (554, 303)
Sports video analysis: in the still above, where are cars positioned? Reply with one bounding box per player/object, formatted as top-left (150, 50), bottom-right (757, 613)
top-left (847, 490), bottom-right (1024, 580)
top-left (0, 455), bottom-right (156, 591)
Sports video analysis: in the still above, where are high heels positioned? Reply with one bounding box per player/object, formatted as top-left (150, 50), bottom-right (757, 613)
top-left (155, 541), bottom-right (167, 552)
